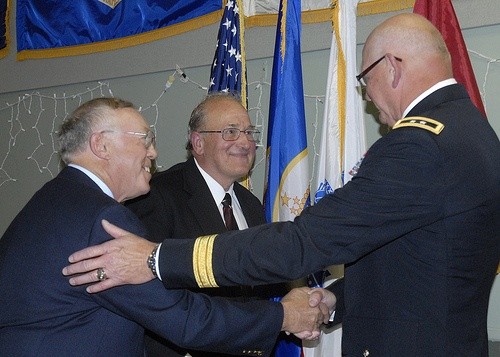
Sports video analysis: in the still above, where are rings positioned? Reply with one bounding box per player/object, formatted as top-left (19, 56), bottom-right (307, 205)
top-left (96, 268), bottom-right (107, 282)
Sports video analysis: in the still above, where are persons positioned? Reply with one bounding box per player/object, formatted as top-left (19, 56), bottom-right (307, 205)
top-left (0, 96), bottom-right (330, 357)
top-left (61, 12), bottom-right (500, 357)
top-left (118, 92), bottom-right (277, 356)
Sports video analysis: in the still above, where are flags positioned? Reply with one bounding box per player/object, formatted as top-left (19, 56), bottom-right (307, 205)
top-left (205, 0), bottom-right (252, 192)
top-left (410, 1), bottom-right (489, 121)
top-left (260, 0), bottom-right (312, 357)
top-left (296, 1), bottom-right (367, 357)
top-left (239, 0), bottom-right (415, 31)
top-left (15, 0), bottom-right (229, 62)
top-left (0, 0), bottom-right (10, 60)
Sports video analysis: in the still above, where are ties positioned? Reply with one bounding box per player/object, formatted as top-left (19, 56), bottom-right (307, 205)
top-left (220, 193), bottom-right (241, 232)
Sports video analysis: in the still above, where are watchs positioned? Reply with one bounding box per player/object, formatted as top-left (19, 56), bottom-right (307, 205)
top-left (147, 244), bottom-right (161, 279)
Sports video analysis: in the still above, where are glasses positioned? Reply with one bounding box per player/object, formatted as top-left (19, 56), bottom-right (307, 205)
top-left (99, 130), bottom-right (156, 149)
top-left (200, 125), bottom-right (261, 143)
top-left (355, 54), bottom-right (402, 90)
